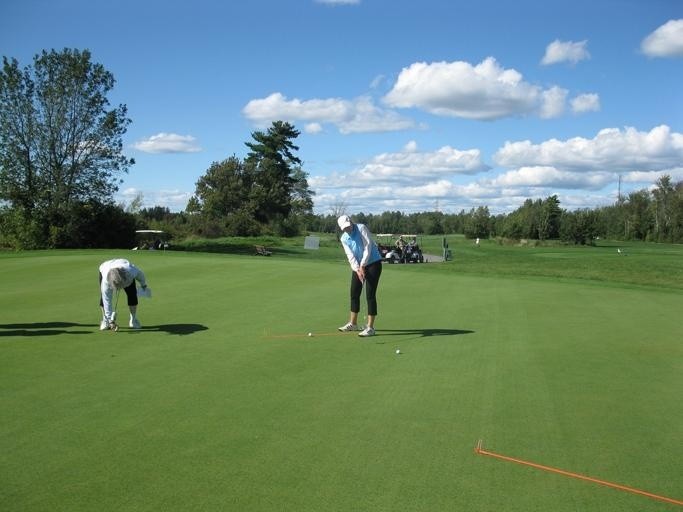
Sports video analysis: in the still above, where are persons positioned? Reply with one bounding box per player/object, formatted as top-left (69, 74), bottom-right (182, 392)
top-left (337, 214), bottom-right (382, 336)
top-left (407, 237), bottom-right (417, 253)
top-left (99, 258), bottom-right (147, 330)
top-left (475, 237), bottom-right (480, 248)
top-left (395, 236), bottom-right (406, 251)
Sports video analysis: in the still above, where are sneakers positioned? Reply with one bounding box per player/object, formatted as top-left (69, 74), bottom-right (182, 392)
top-left (100, 321), bottom-right (110, 331)
top-left (129, 319), bottom-right (141, 328)
top-left (358, 327), bottom-right (376, 336)
top-left (338, 322), bottom-right (359, 331)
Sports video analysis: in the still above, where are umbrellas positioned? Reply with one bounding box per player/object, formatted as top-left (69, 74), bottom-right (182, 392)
top-left (136, 229), bottom-right (164, 241)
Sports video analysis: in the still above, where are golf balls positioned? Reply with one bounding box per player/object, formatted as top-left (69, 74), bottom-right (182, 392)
top-left (395, 349), bottom-right (401, 354)
top-left (308, 331), bottom-right (312, 336)
top-left (114, 326), bottom-right (118, 330)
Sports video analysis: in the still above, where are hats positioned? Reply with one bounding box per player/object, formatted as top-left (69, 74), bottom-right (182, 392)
top-left (337, 215), bottom-right (351, 232)
